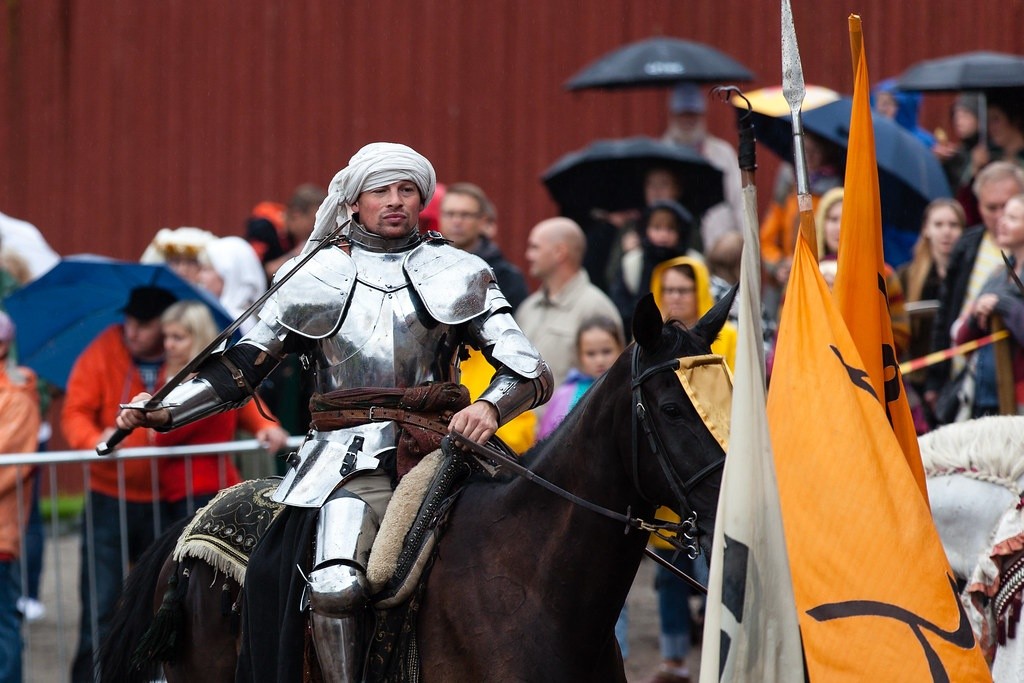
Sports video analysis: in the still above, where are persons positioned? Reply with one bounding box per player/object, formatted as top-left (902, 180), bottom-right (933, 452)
top-left (0, 305), bottom-right (42, 683)
top-left (63, 290), bottom-right (287, 682)
top-left (515, 81), bottom-right (1024, 683)
top-left (115, 142), bottom-right (555, 683)
top-left (0, 231), bottom-right (32, 300)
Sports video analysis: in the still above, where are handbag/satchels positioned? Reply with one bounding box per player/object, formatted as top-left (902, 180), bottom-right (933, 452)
top-left (933, 364), bottom-right (967, 423)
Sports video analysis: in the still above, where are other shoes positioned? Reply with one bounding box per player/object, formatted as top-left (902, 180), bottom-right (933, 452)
top-left (656, 663), bottom-right (691, 683)
top-left (21, 598), bottom-right (46, 623)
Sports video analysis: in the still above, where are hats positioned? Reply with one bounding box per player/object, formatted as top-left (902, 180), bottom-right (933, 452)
top-left (115, 286), bottom-right (179, 323)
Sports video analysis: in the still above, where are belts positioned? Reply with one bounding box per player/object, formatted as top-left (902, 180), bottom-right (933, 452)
top-left (312, 409), bottom-right (452, 435)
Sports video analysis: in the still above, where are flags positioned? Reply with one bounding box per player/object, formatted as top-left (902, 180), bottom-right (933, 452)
top-left (833, 16), bottom-right (932, 518)
top-left (768, 210), bottom-right (996, 682)
top-left (704, 189), bottom-right (805, 683)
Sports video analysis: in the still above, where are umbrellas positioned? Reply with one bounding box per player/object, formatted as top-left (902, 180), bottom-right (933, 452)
top-left (535, 139), bottom-right (726, 215)
top-left (894, 54), bottom-right (1024, 92)
top-left (0, 253), bottom-right (243, 394)
top-left (564, 36), bottom-right (754, 90)
top-left (728, 85), bottom-right (954, 202)
top-left (0, 210), bottom-right (61, 282)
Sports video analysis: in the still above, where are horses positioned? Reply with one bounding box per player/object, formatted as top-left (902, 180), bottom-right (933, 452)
top-left (915, 413), bottom-right (1024, 683)
top-left (104, 283), bottom-right (759, 683)
